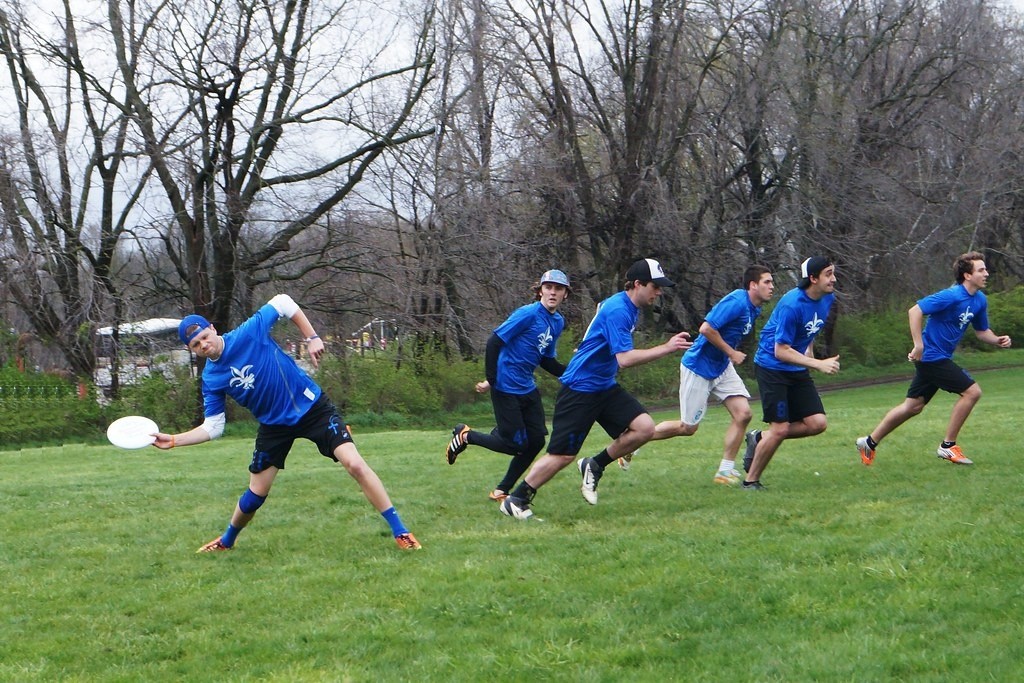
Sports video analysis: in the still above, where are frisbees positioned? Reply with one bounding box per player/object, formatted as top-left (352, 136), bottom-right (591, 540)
top-left (106, 415), bottom-right (160, 449)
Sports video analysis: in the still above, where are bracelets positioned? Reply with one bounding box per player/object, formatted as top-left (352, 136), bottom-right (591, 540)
top-left (305, 335), bottom-right (319, 342)
top-left (171, 434), bottom-right (174, 448)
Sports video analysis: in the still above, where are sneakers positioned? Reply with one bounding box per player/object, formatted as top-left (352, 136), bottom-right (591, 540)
top-left (937, 442), bottom-right (974, 464)
top-left (195, 535), bottom-right (239, 554)
top-left (445, 423), bottom-right (473, 466)
top-left (617, 449), bottom-right (639, 471)
top-left (712, 469), bottom-right (742, 489)
top-left (499, 487), bottom-right (544, 524)
top-left (743, 429), bottom-right (762, 473)
top-left (856, 436), bottom-right (876, 466)
top-left (577, 457), bottom-right (605, 505)
top-left (488, 489), bottom-right (510, 502)
top-left (741, 481), bottom-right (768, 492)
top-left (395, 532), bottom-right (422, 552)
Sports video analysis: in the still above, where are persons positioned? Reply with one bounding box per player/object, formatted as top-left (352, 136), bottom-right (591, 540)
top-left (445, 270), bottom-right (571, 505)
top-left (741, 256), bottom-right (841, 492)
top-left (617, 265), bottom-right (773, 483)
top-left (148, 294), bottom-right (421, 552)
top-left (856, 251), bottom-right (1011, 465)
top-left (499, 258), bottom-right (694, 522)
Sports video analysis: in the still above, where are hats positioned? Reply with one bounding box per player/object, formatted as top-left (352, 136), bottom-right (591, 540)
top-left (624, 258), bottom-right (678, 288)
top-left (799, 256), bottom-right (828, 288)
top-left (541, 269), bottom-right (570, 287)
top-left (178, 314), bottom-right (211, 346)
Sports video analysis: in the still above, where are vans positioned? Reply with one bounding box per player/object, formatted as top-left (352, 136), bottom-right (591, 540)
top-left (93, 318), bottom-right (198, 410)
